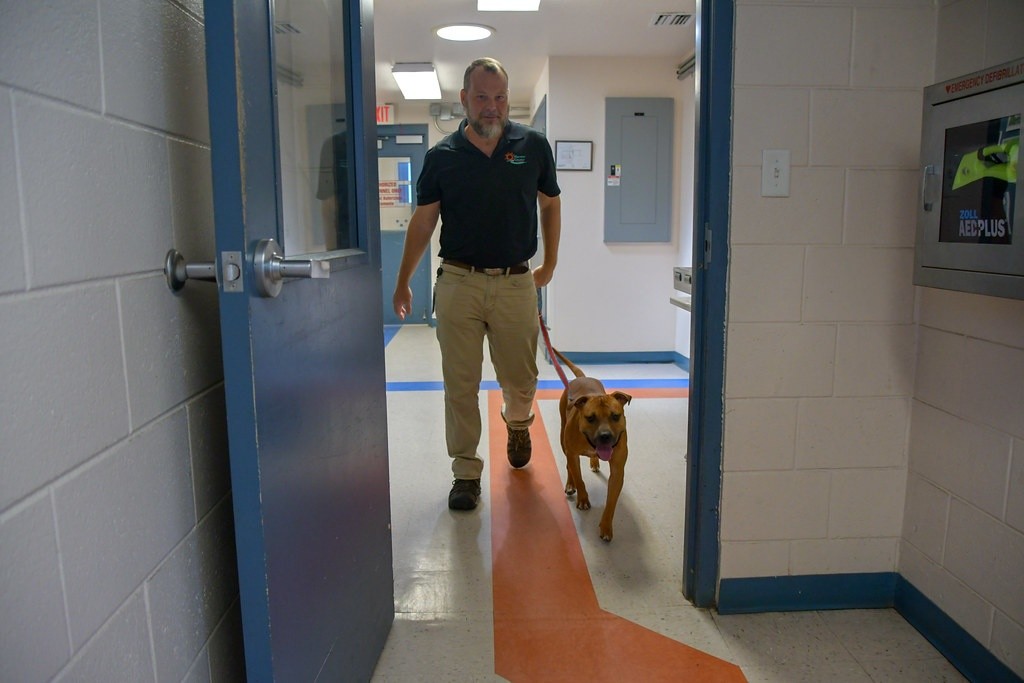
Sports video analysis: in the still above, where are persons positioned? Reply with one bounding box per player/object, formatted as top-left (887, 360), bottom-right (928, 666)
top-left (392, 58), bottom-right (562, 510)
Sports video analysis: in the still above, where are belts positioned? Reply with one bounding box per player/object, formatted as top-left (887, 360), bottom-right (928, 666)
top-left (442, 258), bottom-right (530, 275)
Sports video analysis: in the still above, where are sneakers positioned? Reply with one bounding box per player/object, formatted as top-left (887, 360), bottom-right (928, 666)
top-left (506, 425), bottom-right (532, 469)
top-left (448, 477), bottom-right (481, 511)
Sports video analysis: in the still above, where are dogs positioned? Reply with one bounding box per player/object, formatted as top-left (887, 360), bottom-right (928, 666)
top-left (552, 346), bottom-right (633, 543)
